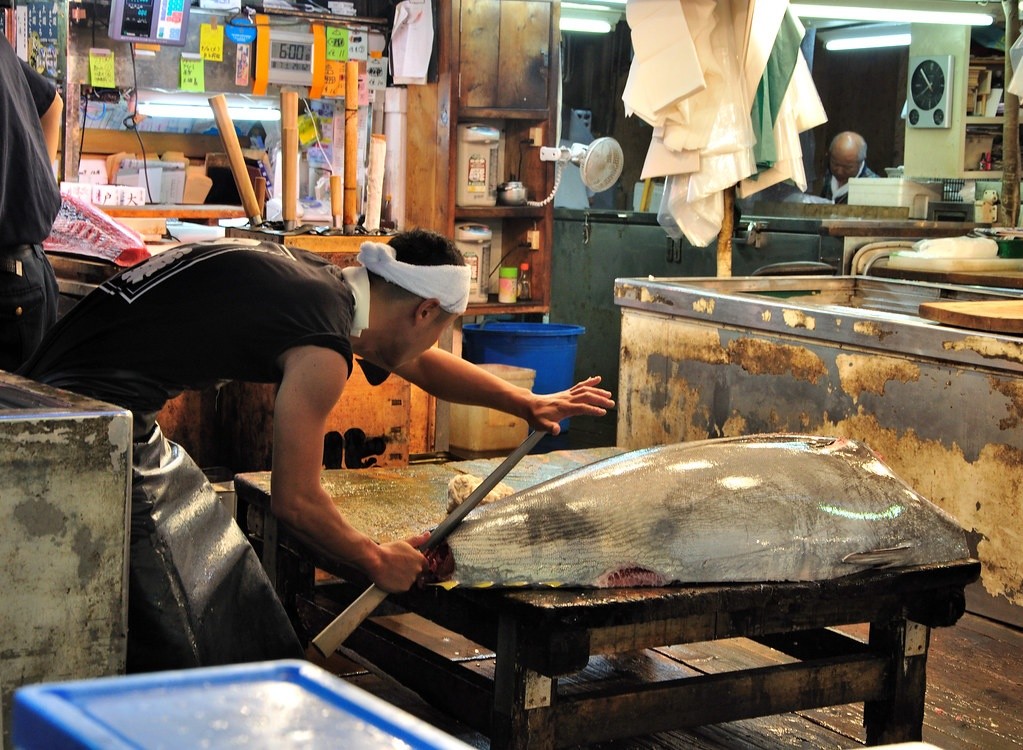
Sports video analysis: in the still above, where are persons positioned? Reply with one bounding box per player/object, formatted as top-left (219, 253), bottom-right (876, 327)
top-left (28, 227), bottom-right (615, 672)
top-left (821, 132), bottom-right (879, 205)
top-left (0, 34), bottom-right (63, 374)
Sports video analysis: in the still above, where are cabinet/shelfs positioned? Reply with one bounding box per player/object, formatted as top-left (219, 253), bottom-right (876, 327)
top-left (428, 0), bottom-right (564, 455)
top-left (903, 22), bottom-right (1023, 180)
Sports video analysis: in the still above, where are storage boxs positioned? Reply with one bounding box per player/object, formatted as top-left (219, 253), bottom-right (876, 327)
top-left (15, 658), bottom-right (481, 750)
top-left (449, 364), bottom-right (537, 453)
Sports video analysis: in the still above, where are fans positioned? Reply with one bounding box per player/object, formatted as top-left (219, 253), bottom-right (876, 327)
top-left (540, 134), bottom-right (624, 192)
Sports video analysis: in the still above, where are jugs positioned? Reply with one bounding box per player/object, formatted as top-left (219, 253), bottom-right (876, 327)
top-left (497, 174), bottom-right (526, 206)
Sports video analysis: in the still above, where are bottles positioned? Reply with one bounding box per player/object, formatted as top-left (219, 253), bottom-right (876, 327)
top-left (517, 263), bottom-right (532, 300)
top-left (498, 266), bottom-right (518, 303)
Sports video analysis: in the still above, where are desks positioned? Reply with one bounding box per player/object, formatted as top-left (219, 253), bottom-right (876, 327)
top-left (230, 444), bottom-right (989, 750)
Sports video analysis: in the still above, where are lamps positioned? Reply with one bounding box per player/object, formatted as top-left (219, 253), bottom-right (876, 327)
top-left (132, 100), bottom-right (281, 123)
top-left (789, 3), bottom-right (994, 27)
top-left (553, 13), bottom-right (613, 35)
top-left (823, 32), bottom-right (912, 51)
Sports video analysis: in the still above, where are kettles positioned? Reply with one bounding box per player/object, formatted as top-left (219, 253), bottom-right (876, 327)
top-left (457, 122), bottom-right (500, 207)
top-left (454, 222), bottom-right (492, 303)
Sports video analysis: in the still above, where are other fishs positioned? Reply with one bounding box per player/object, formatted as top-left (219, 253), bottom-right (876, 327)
top-left (415, 433), bottom-right (971, 592)
top-left (43, 189), bottom-right (151, 267)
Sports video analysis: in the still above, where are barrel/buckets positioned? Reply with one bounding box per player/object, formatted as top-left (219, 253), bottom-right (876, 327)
top-left (462, 323), bottom-right (586, 434)
top-left (449, 363), bottom-right (536, 451)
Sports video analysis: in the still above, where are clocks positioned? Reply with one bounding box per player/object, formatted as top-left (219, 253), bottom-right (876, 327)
top-left (906, 54), bottom-right (954, 129)
